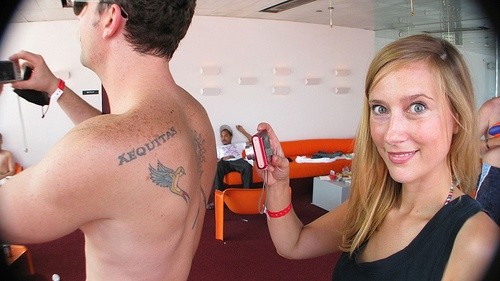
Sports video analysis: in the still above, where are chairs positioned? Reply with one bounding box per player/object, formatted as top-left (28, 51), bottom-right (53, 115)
top-left (214, 186), bottom-right (291, 242)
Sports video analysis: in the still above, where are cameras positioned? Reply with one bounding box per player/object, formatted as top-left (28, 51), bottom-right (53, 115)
top-left (0, 60), bottom-right (22, 84)
top-left (252, 129), bottom-right (273, 169)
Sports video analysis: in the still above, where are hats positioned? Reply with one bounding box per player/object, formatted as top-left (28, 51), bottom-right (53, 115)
top-left (219, 124), bottom-right (233, 136)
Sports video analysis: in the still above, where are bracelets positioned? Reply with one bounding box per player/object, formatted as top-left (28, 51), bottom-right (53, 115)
top-left (51, 77), bottom-right (65, 104)
top-left (264, 201), bottom-right (292, 218)
top-left (485, 139), bottom-right (490, 150)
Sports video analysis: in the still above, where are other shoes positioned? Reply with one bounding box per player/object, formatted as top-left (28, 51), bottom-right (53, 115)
top-left (207, 203), bottom-right (215, 209)
top-left (241, 216), bottom-right (249, 222)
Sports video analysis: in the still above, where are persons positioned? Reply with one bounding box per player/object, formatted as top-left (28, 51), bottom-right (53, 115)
top-left (257, 34), bottom-right (500, 281)
top-left (206, 123), bottom-right (252, 223)
top-left (0, 0), bottom-right (218, 281)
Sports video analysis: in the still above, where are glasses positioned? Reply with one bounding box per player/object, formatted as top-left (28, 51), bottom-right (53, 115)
top-left (73, 0), bottom-right (127, 18)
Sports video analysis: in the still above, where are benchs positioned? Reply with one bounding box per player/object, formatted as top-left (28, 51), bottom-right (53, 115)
top-left (221, 139), bottom-right (356, 185)
